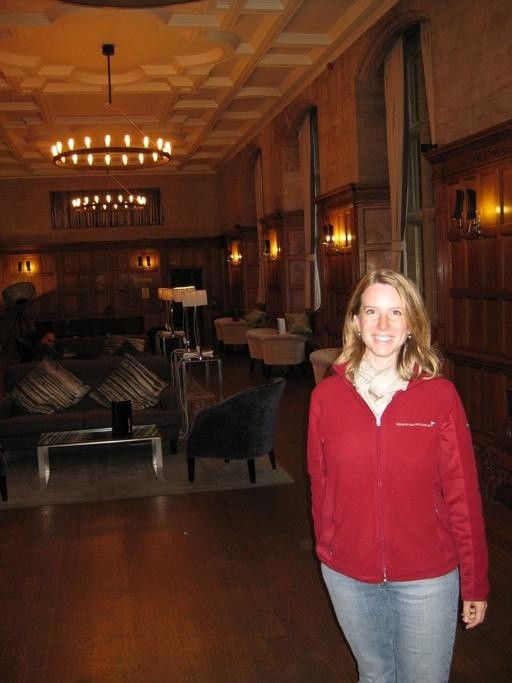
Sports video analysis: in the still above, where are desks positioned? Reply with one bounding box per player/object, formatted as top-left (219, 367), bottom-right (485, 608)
top-left (169, 345), bottom-right (226, 438)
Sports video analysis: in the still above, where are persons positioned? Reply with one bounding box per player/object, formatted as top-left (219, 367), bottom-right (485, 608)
top-left (307, 268), bottom-right (491, 682)
top-left (31, 331), bottom-right (55, 362)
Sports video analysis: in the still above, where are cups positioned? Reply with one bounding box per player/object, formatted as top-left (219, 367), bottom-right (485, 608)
top-left (276, 317), bottom-right (286, 334)
top-left (111, 398), bottom-right (133, 437)
top-left (232, 310), bottom-right (240, 321)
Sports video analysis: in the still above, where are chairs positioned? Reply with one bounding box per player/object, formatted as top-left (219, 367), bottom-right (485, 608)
top-left (214, 314), bottom-right (344, 389)
top-left (188, 372), bottom-right (286, 487)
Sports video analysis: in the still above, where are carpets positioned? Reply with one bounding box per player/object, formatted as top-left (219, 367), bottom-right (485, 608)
top-left (0, 449), bottom-right (292, 507)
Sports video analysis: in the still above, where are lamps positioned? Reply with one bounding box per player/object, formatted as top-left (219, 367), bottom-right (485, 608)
top-left (321, 223), bottom-right (336, 255)
top-left (45, 99), bottom-right (174, 171)
top-left (69, 168), bottom-right (147, 218)
top-left (261, 238), bottom-right (273, 262)
top-left (137, 254), bottom-right (151, 272)
top-left (451, 185), bottom-right (484, 239)
top-left (158, 284), bottom-right (211, 357)
top-left (225, 249), bottom-right (235, 264)
top-left (16, 259), bottom-right (32, 275)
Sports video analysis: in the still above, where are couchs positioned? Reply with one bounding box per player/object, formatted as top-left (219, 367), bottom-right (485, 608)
top-left (0, 313), bottom-right (178, 467)
